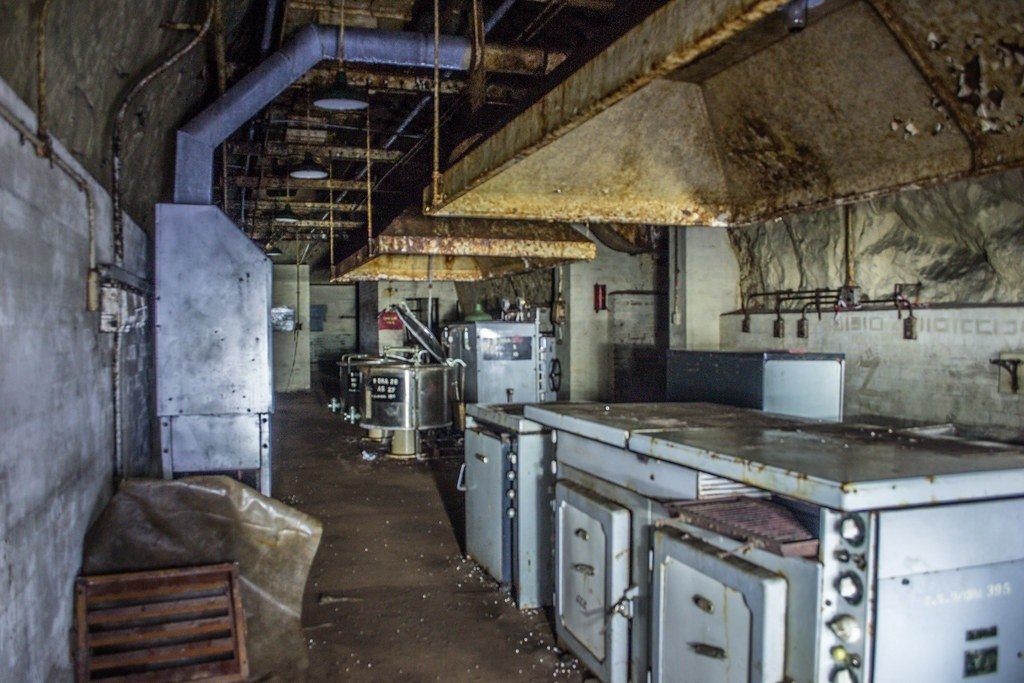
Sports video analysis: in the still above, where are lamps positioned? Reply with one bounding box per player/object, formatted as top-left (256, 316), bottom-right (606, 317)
top-left (290, 80), bottom-right (329, 181)
top-left (311, 0), bottom-right (369, 114)
top-left (274, 153), bottom-right (298, 224)
